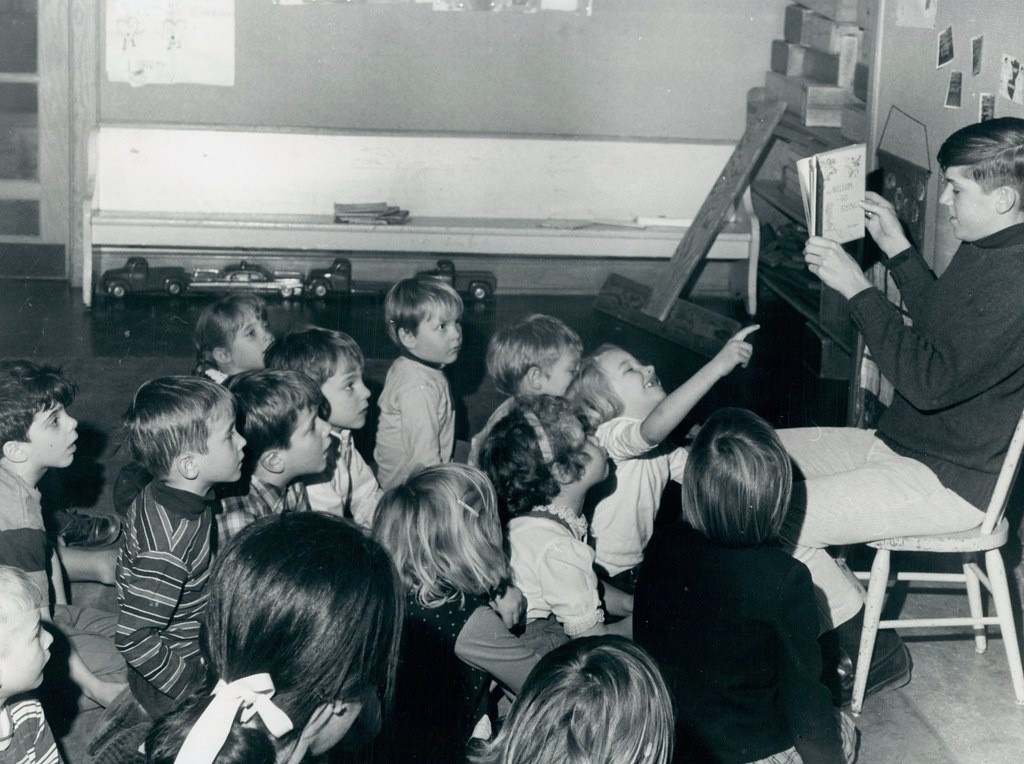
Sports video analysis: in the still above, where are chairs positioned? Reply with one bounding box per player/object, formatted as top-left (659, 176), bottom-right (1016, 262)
top-left (845, 410), bottom-right (1024, 718)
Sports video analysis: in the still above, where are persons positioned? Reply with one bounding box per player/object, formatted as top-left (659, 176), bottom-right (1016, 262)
top-left (372, 276), bottom-right (465, 493)
top-left (632, 407), bottom-right (860, 763)
top-left (560, 322), bottom-right (760, 593)
top-left (461, 313), bottom-right (584, 471)
top-left (485, 392), bottom-right (635, 658)
top-left (208, 367), bottom-right (334, 560)
top-left (0, 358), bottom-right (131, 714)
top-left (92, 509), bottom-right (407, 764)
top-left (190, 290), bottom-right (277, 385)
top-left (756, 115), bottom-right (1024, 714)
top-left (86, 373), bottom-right (247, 764)
top-left (369, 462), bottom-right (540, 763)
top-left (262, 326), bottom-right (389, 540)
top-left (0, 564), bottom-right (67, 764)
top-left (463, 630), bottom-right (675, 763)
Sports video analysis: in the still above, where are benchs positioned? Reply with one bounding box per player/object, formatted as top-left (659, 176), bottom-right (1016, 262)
top-left (83, 122), bottom-right (760, 316)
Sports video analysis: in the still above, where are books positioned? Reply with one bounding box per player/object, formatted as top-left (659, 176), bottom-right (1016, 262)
top-left (332, 200), bottom-right (409, 227)
top-left (796, 142), bottom-right (866, 247)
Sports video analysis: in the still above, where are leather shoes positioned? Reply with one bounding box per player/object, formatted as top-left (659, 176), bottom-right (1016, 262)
top-left (822, 637), bottom-right (911, 710)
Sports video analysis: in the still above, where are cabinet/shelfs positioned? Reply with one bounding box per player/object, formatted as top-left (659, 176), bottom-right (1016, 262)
top-left (745, 93), bottom-right (869, 380)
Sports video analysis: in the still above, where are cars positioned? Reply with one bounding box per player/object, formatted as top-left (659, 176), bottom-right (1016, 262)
top-left (188, 260), bottom-right (304, 301)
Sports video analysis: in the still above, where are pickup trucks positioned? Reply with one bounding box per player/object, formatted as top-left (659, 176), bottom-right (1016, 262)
top-left (100, 257), bottom-right (188, 299)
